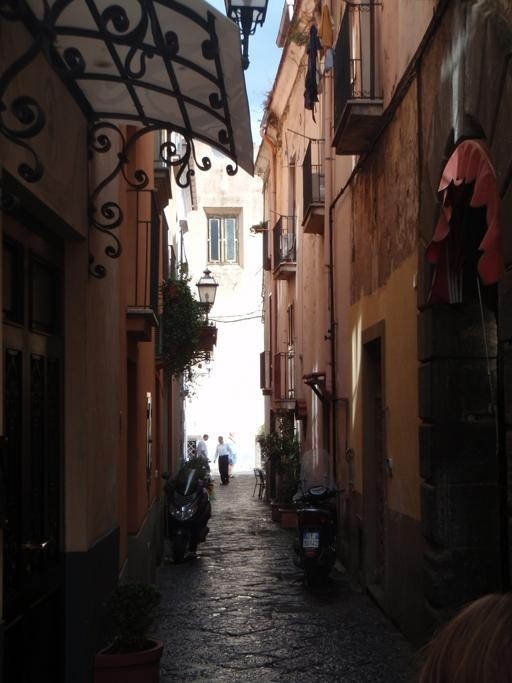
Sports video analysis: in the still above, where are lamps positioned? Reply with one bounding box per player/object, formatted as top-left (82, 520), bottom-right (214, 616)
top-left (179, 221), bottom-right (219, 323)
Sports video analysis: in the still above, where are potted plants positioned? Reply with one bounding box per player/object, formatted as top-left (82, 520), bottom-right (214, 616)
top-left (256, 405), bottom-right (305, 529)
top-left (152, 273), bottom-right (215, 404)
top-left (94, 581), bottom-right (165, 683)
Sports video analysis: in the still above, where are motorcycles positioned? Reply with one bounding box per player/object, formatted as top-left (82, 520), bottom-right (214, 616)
top-left (293, 484), bottom-right (346, 587)
top-left (162, 468), bottom-right (211, 562)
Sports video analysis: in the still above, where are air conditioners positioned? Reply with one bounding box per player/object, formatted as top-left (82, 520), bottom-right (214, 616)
top-left (279, 233), bottom-right (294, 261)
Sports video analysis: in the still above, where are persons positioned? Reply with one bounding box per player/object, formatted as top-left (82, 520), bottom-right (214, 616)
top-left (226, 431), bottom-right (237, 478)
top-left (197, 433), bottom-right (214, 482)
top-left (417, 589), bottom-right (510, 683)
top-left (213, 435), bottom-right (233, 485)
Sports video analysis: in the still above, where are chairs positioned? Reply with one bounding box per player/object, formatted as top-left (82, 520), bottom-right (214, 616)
top-left (252, 468), bottom-right (266, 500)
